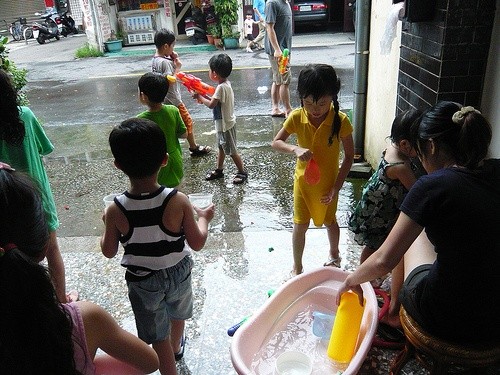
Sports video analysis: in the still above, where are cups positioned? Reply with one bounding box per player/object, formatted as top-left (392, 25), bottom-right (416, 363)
top-left (102, 194), bottom-right (122, 209)
top-left (187, 194), bottom-right (214, 215)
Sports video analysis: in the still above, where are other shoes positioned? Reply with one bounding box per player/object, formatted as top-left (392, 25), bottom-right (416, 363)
top-left (324, 257), bottom-right (341, 267)
top-left (174, 334), bottom-right (185, 358)
top-left (67, 289), bottom-right (79, 304)
top-left (282, 268), bottom-right (303, 283)
top-left (246, 47), bottom-right (254, 53)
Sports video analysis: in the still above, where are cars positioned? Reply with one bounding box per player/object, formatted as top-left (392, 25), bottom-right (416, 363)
top-left (293, 0), bottom-right (331, 27)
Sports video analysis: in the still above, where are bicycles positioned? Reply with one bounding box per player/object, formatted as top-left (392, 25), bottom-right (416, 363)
top-left (9, 17), bottom-right (32, 41)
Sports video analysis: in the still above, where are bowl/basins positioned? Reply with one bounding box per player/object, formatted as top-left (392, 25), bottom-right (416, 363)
top-left (273, 350), bottom-right (313, 375)
top-left (312, 312), bottom-right (336, 340)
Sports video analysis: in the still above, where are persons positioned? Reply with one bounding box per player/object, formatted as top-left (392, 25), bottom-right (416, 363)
top-left (245, 0), bottom-right (269, 52)
top-left (137, 28), bottom-right (247, 188)
top-left (100, 118), bottom-right (214, 375)
top-left (336, 100), bottom-right (500, 340)
top-left (0, 69), bottom-right (80, 305)
top-left (0, 161), bottom-right (161, 375)
top-left (271, 65), bottom-right (354, 277)
top-left (264, 0), bottom-right (297, 119)
top-left (349, 110), bottom-right (423, 327)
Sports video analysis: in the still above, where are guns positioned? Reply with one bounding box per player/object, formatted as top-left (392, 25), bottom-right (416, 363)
top-left (274, 48), bottom-right (289, 75)
top-left (167, 72), bottom-right (216, 104)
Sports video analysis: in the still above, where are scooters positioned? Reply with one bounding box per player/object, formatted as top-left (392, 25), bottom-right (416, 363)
top-left (184, 2), bottom-right (217, 46)
top-left (30, 8), bottom-right (60, 44)
top-left (53, 3), bottom-right (79, 37)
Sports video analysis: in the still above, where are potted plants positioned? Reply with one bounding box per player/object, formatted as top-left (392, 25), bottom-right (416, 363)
top-left (206, 0), bottom-right (240, 49)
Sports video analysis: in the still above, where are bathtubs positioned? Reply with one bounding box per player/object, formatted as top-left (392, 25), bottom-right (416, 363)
top-left (229, 266), bottom-right (380, 375)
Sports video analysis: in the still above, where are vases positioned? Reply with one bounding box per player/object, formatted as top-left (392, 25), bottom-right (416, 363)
top-left (104, 39), bottom-right (124, 52)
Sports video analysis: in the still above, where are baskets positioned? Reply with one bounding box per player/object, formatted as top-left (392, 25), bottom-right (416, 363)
top-left (19, 18), bottom-right (26, 24)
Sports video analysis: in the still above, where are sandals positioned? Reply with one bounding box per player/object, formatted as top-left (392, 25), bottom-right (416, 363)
top-left (206, 169), bottom-right (224, 180)
top-left (189, 144), bottom-right (212, 157)
top-left (233, 172), bottom-right (248, 184)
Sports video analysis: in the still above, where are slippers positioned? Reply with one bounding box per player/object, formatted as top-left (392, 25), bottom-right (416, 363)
top-left (272, 113), bottom-right (285, 117)
top-left (378, 322), bottom-right (405, 342)
